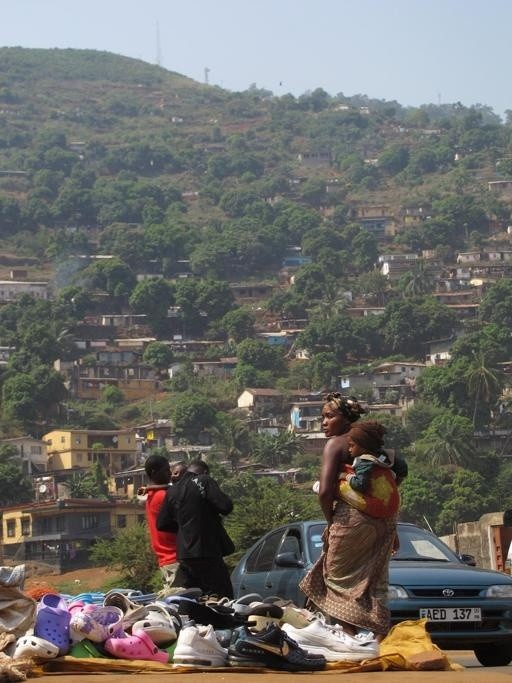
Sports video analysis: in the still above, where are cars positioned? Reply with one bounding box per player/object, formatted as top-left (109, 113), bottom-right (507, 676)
top-left (231, 521), bottom-right (512, 667)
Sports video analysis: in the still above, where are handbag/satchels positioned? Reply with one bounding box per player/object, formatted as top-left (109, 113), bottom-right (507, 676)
top-left (215, 518), bottom-right (235, 556)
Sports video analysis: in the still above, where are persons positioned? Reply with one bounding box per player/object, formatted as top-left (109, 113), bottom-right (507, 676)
top-left (136, 451), bottom-right (236, 600)
top-left (299, 391), bottom-right (409, 642)
